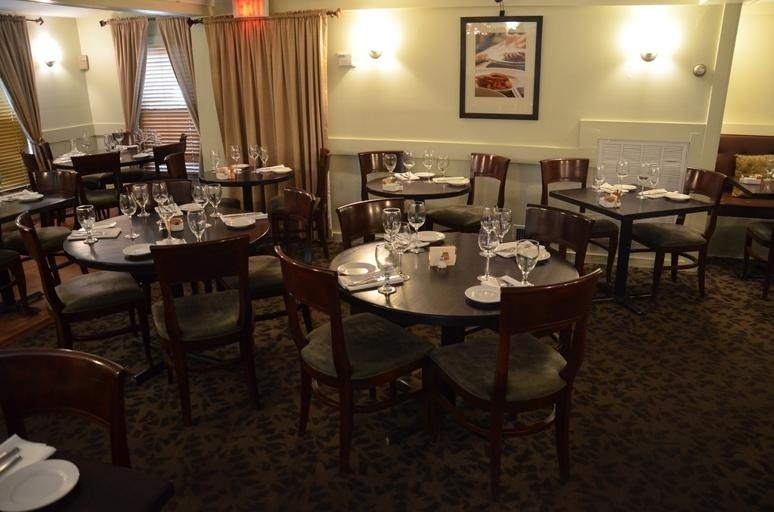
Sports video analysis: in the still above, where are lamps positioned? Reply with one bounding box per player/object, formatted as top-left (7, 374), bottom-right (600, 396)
top-left (166, 153), bottom-right (189, 181)
top-left (723, 131), bottom-right (770, 221)
top-left (72, 150), bottom-right (126, 221)
top-left (522, 204), bottom-right (593, 358)
top-left (742, 220), bottom-right (774, 299)
top-left (540, 158), bottom-right (619, 286)
top-left (156, 143), bottom-right (185, 179)
top-left (426, 153), bottom-right (511, 234)
top-left (0, 347), bottom-right (131, 472)
top-left (275, 247), bottom-right (435, 465)
top-left (22, 152), bottom-right (45, 223)
top-left (1, 250), bottom-right (29, 315)
top-left (41, 141), bottom-right (55, 172)
top-left (3, 170), bottom-right (88, 317)
top-left (270, 148), bottom-right (332, 257)
top-left (338, 198), bottom-right (396, 313)
top-left (630, 168), bottom-right (729, 304)
top-left (358, 151), bottom-right (407, 243)
top-left (17, 211), bottom-right (149, 355)
top-left (147, 180), bottom-right (194, 212)
top-left (427, 269), bottom-right (603, 498)
top-left (219, 190), bottom-right (320, 330)
top-left (153, 237), bottom-right (263, 423)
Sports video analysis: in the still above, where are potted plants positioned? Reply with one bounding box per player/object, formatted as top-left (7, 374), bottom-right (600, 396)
top-left (458, 15), bottom-right (542, 121)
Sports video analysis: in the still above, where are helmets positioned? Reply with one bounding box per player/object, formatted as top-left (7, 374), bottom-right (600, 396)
top-left (538, 251), bottom-right (551, 261)
top-left (665, 193), bottom-right (690, 201)
top-left (231, 164), bottom-right (250, 169)
top-left (225, 218), bottom-right (257, 228)
top-left (465, 285), bottom-right (501, 303)
top-left (132, 153), bottom-right (149, 159)
top-left (447, 180), bottom-right (470, 186)
top-left (0, 459), bottom-right (80, 512)
top-left (413, 231), bottom-right (446, 243)
top-left (415, 173), bottom-right (436, 178)
top-left (17, 194), bottom-right (44, 203)
top-left (122, 243), bottom-right (155, 256)
top-left (337, 262), bottom-right (376, 275)
top-left (614, 184), bottom-right (637, 190)
top-left (272, 167), bottom-right (293, 175)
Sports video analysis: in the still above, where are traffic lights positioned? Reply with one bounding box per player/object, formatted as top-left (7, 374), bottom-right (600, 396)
top-left (732, 154), bottom-right (774, 196)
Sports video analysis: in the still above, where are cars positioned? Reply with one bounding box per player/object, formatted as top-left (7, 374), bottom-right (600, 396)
top-left (170, 218), bottom-right (184, 231)
top-left (179, 203), bottom-right (203, 212)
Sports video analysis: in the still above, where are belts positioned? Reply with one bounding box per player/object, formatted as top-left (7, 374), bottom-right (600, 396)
top-left (438, 152), bottom-right (456, 178)
top-left (209, 149), bottom-right (219, 175)
top-left (119, 191), bottom-right (140, 240)
top-left (408, 201), bottom-right (426, 253)
top-left (402, 151), bottom-right (415, 183)
top-left (114, 129), bottom-right (124, 153)
top-left (158, 195), bottom-right (176, 243)
top-left (81, 131), bottom-right (93, 154)
top-left (384, 154), bottom-right (397, 184)
top-left (134, 128), bottom-right (144, 153)
top-left (649, 163), bottom-right (661, 199)
top-left (187, 208), bottom-right (207, 242)
top-left (248, 144), bottom-right (259, 174)
top-left (194, 183), bottom-right (212, 228)
top-left (423, 150), bottom-right (434, 183)
top-left (636, 162), bottom-right (649, 200)
top-left (382, 208), bottom-right (401, 251)
top-left (207, 184), bottom-right (222, 218)
top-left (230, 144), bottom-right (242, 174)
top-left (594, 162), bottom-right (606, 194)
top-left (375, 243), bottom-right (398, 294)
top-left (258, 145), bottom-right (269, 173)
top-left (75, 205), bottom-right (97, 247)
top-left (478, 221), bottom-right (499, 280)
top-left (762, 169), bottom-right (773, 192)
top-left (392, 222), bottom-right (412, 280)
top-left (152, 180), bottom-right (168, 205)
top-left (132, 183), bottom-right (150, 216)
top-left (481, 207), bottom-right (497, 242)
top-left (616, 159), bottom-right (630, 195)
top-left (493, 208), bottom-right (512, 243)
top-left (515, 239), bottom-right (540, 285)
top-left (104, 135), bottom-right (113, 153)
